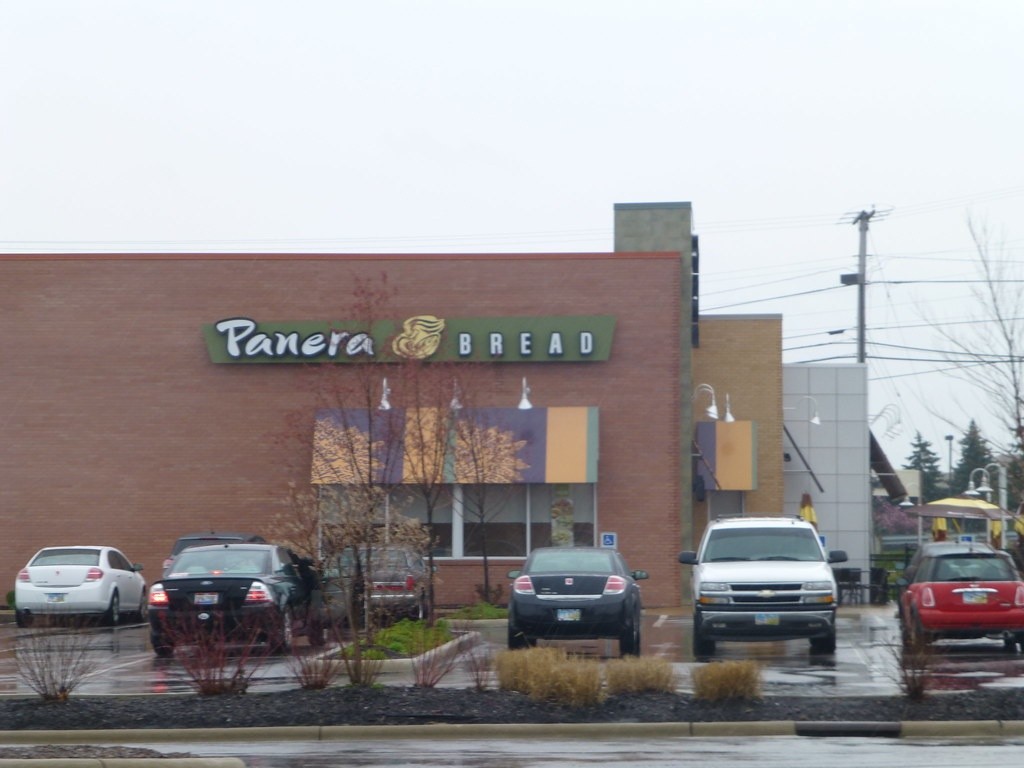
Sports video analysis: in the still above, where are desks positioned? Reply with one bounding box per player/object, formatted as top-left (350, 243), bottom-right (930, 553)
top-left (850, 570), bottom-right (896, 604)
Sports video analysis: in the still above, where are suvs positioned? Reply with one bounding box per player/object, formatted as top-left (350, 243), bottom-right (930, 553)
top-left (680, 514), bottom-right (849, 657)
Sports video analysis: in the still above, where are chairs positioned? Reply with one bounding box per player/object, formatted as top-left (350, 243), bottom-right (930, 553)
top-left (831, 566), bottom-right (890, 605)
top-left (937, 561), bottom-right (962, 579)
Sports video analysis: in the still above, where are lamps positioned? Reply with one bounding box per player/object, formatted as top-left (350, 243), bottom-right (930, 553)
top-left (696, 382), bottom-right (718, 419)
top-left (781, 396), bottom-right (822, 425)
top-left (722, 391), bottom-right (737, 424)
top-left (448, 376), bottom-right (462, 412)
top-left (376, 376), bottom-right (395, 413)
top-left (866, 402), bottom-right (906, 443)
top-left (517, 377), bottom-right (535, 412)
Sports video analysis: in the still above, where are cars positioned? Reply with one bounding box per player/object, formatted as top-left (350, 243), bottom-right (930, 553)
top-left (900, 542), bottom-right (1024, 655)
top-left (314, 542), bottom-right (428, 626)
top-left (148, 542), bottom-right (328, 657)
top-left (13, 547), bottom-right (151, 627)
top-left (165, 533), bottom-right (268, 572)
top-left (507, 543), bottom-right (648, 659)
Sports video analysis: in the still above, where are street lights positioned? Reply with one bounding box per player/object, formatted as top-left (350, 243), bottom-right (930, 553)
top-left (944, 434), bottom-right (954, 496)
top-left (963, 467), bottom-right (991, 545)
top-left (977, 462), bottom-right (1007, 553)
